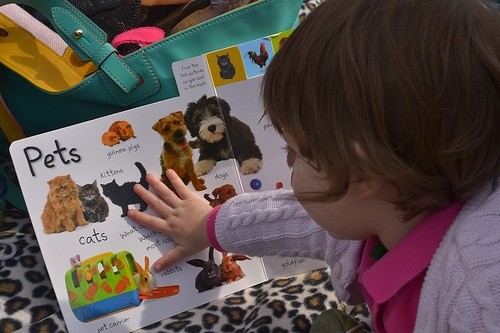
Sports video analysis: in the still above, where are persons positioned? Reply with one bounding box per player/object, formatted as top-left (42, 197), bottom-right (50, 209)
top-left (126, 0), bottom-right (499, 333)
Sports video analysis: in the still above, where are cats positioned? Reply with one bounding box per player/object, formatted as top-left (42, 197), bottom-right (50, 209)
top-left (216, 53), bottom-right (235, 80)
top-left (75, 179), bottom-right (109, 224)
top-left (40, 173), bottom-right (90, 234)
top-left (100, 161), bottom-right (149, 218)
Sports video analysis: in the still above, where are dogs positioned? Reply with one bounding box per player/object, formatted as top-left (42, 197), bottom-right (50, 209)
top-left (183, 95), bottom-right (263, 178)
top-left (151, 110), bottom-right (208, 199)
top-left (204, 184), bottom-right (238, 208)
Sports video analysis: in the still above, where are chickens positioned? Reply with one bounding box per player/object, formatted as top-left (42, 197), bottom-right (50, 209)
top-left (247, 42), bottom-right (269, 71)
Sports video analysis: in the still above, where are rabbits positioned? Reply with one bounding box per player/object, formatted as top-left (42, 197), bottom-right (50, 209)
top-left (185, 246), bottom-right (224, 293)
top-left (219, 250), bottom-right (252, 284)
top-left (130, 256), bottom-right (162, 296)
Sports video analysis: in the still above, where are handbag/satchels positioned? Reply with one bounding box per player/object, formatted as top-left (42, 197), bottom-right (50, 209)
top-left (0, 1), bottom-right (303, 212)
